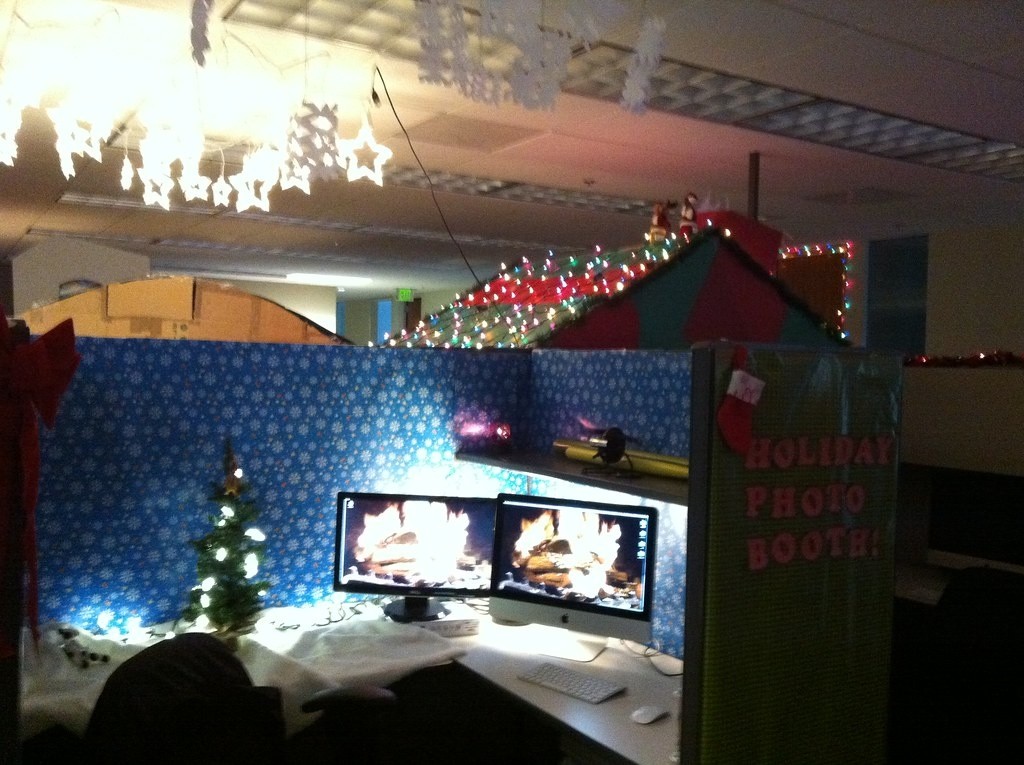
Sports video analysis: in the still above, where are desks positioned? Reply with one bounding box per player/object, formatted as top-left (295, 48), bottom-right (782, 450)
top-left (20, 598), bottom-right (685, 765)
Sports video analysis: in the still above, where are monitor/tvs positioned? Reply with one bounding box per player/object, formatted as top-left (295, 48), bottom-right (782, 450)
top-left (489, 493), bottom-right (658, 663)
top-left (333, 492), bottom-right (498, 624)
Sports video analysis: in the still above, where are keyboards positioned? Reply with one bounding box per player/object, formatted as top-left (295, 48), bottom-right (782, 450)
top-left (517, 661), bottom-right (627, 705)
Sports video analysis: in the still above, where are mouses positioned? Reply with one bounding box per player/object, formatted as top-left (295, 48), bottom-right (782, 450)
top-left (630, 705), bottom-right (668, 725)
